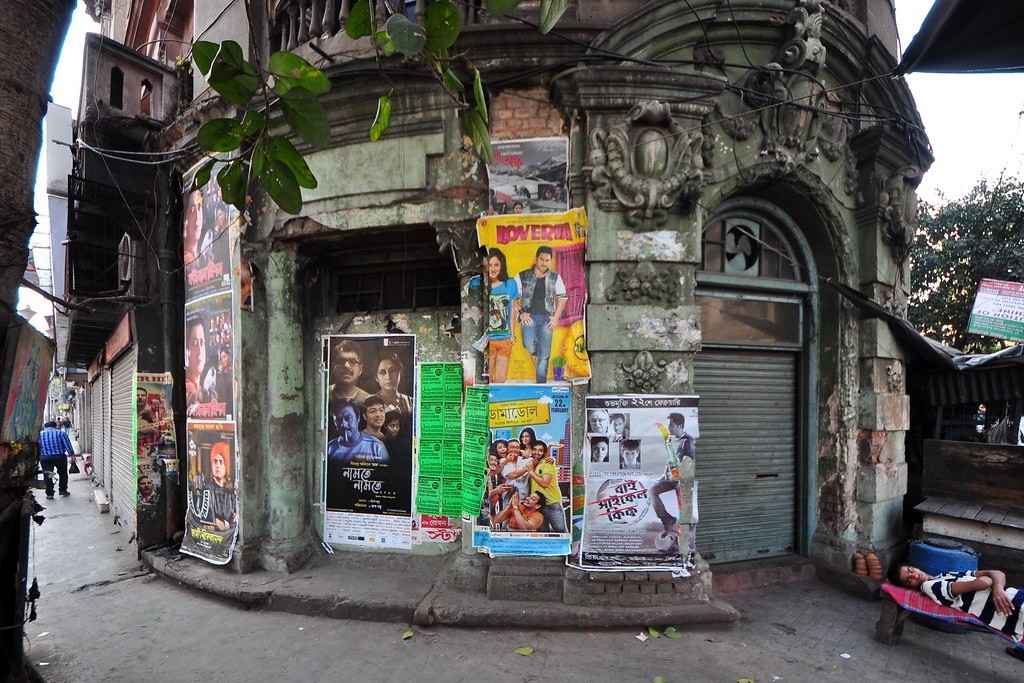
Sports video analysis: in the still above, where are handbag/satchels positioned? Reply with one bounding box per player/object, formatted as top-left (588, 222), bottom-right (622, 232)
top-left (69, 462), bottom-right (80, 474)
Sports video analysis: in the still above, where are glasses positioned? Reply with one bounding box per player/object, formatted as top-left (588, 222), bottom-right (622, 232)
top-left (211, 458), bottom-right (225, 465)
top-left (335, 358), bottom-right (361, 367)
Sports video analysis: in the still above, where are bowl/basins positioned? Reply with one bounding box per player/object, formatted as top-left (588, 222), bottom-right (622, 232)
top-left (853, 552), bottom-right (883, 580)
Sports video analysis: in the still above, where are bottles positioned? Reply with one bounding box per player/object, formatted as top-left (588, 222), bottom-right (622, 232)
top-left (665, 443), bottom-right (680, 479)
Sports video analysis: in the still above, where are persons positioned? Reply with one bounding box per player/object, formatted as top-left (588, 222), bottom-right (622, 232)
top-left (138, 477), bottom-right (157, 503)
top-left (182, 175), bottom-right (230, 271)
top-left (75, 427), bottom-right (79, 441)
top-left (888, 562), bottom-right (1024, 644)
top-left (485, 427), bottom-right (568, 532)
top-left (185, 312), bottom-right (234, 419)
top-left (137, 387), bottom-right (168, 439)
top-left (55, 416), bottom-right (71, 435)
top-left (588, 409), bottom-right (640, 470)
top-left (649, 413), bottom-right (695, 539)
top-left (327, 338), bottom-right (413, 494)
top-left (488, 248), bottom-right (518, 384)
top-left (488, 182), bottom-right (567, 215)
top-left (515, 247), bottom-right (568, 384)
top-left (39, 422), bottom-right (76, 499)
top-left (189, 442), bottom-right (236, 532)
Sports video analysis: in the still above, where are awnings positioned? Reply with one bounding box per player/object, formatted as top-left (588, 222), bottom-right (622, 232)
top-left (895, 0), bottom-right (1024, 74)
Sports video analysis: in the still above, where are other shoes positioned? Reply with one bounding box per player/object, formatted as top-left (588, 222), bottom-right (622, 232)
top-left (59, 490), bottom-right (71, 495)
top-left (46, 495), bottom-right (53, 499)
top-left (1006, 646), bottom-right (1024, 662)
top-left (661, 517), bottom-right (676, 539)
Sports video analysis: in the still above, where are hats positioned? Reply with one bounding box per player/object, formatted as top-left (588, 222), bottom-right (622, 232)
top-left (45, 422), bottom-right (57, 428)
top-left (211, 442), bottom-right (230, 473)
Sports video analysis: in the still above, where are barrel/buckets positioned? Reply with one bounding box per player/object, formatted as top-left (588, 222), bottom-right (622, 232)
top-left (908, 536), bottom-right (980, 634)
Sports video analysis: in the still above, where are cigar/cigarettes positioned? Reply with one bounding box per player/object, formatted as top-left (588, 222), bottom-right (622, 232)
top-left (344, 430), bottom-right (348, 441)
